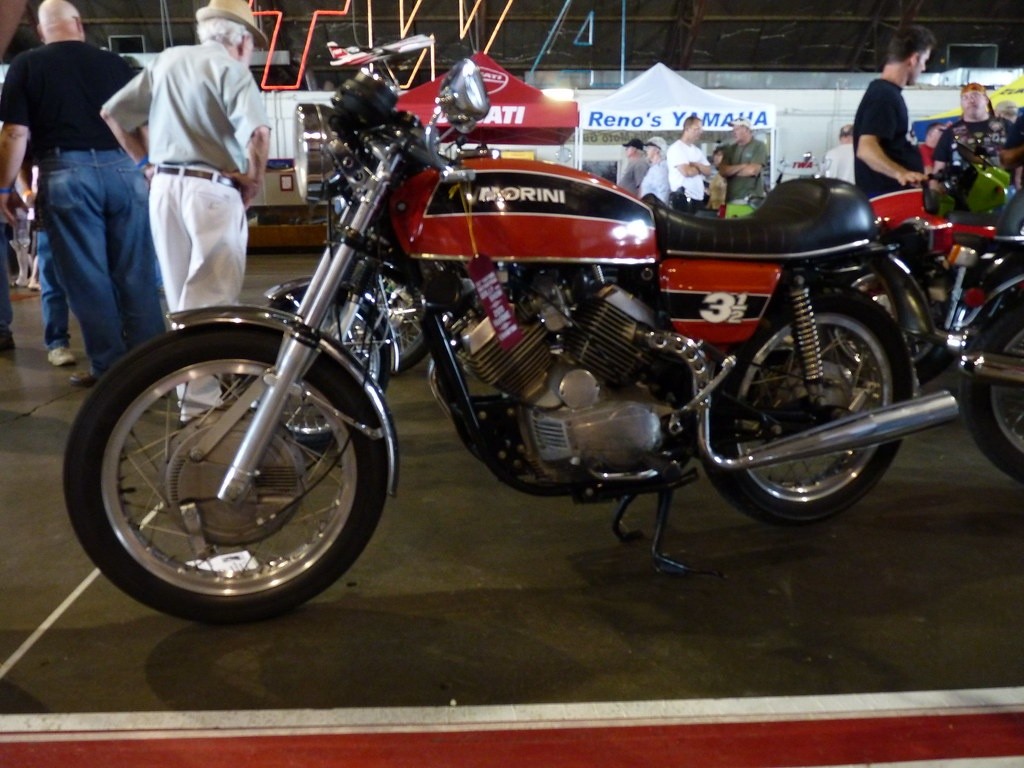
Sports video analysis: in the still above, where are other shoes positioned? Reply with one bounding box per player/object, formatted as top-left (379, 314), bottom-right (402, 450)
top-left (69, 370), bottom-right (96, 386)
top-left (47, 345), bottom-right (76, 367)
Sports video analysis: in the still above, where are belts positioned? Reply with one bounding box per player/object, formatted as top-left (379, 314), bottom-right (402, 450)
top-left (157, 167), bottom-right (241, 189)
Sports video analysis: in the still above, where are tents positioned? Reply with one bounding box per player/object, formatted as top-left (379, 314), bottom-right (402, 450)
top-left (910, 74), bottom-right (1024, 141)
top-left (389, 51), bottom-right (777, 192)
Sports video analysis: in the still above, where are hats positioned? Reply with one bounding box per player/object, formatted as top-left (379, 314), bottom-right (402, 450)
top-left (644, 136), bottom-right (667, 151)
top-left (195, 0), bottom-right (269, 51)
top-left (623, 138), bottom-right (644, 151)
top-left (729, 117), bottom-right (753, 131)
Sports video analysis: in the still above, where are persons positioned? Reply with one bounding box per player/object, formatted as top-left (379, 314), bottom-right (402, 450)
top-left (0, 0), bottom-right (274, 426)
top-left (828, 27), bottom-right (1024, 302)
top-left (611, 117), bottom-right (769, 214)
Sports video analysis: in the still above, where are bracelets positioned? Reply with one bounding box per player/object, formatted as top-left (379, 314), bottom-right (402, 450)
top-left (137, 155), bottom-right (148, 169)
top-left (1, 189), bottom-right (11, 193)
top-left (23, 190), bottom-right (31, 204)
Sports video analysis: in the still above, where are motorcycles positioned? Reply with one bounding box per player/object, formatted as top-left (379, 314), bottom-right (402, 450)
top-left (61, 56), bottom-right (959, 624)
top-left (826, 127), bottom-right (1000, 391)
top-left (945, 186), bottom-right (1023, 484)
